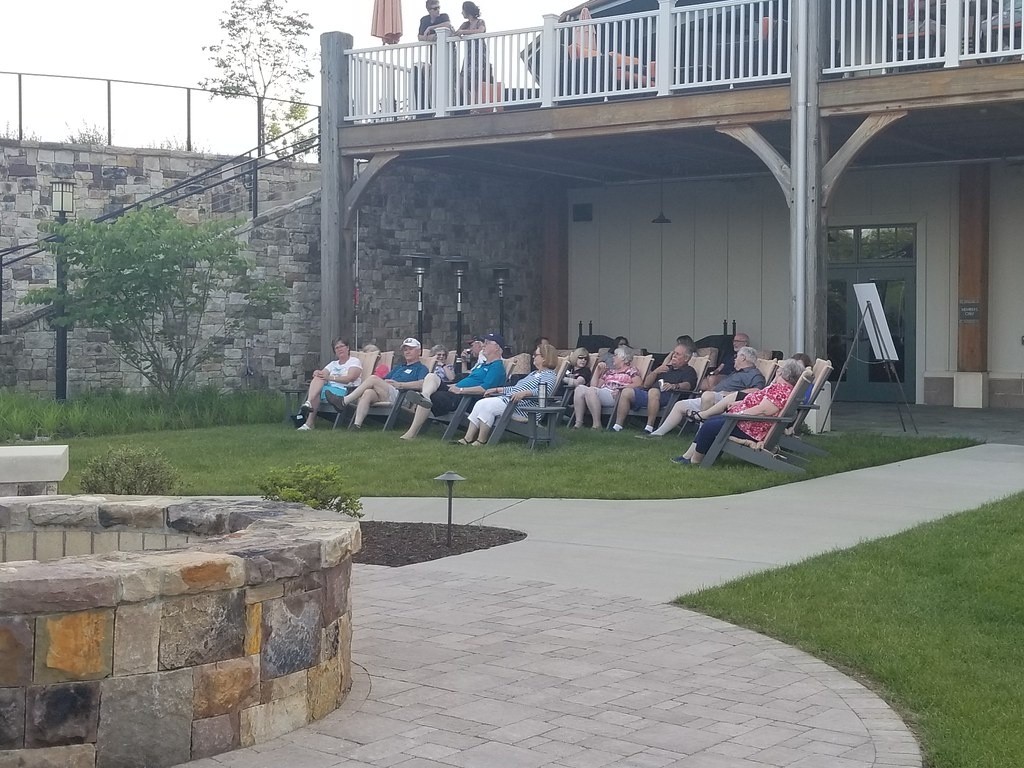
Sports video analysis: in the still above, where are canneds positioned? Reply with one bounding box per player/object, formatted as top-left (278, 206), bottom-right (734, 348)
top-left (658, 379), bottom-right (665, 391)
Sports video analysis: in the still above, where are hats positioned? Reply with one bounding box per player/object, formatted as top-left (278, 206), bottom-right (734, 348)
top-left (468, 335), bottom-right (485, 344)
top-left (401, 338), bottom-right (420, 349)
top-left (484, 333), bottom-right (504, 350)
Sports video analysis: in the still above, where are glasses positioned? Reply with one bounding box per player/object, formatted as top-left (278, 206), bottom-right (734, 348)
top-left (578, 357), bottom-right (588, 360)
top-left (533, 352), bottom-right (541, 358)
top-left (732, 340), bottom-right (745, 342)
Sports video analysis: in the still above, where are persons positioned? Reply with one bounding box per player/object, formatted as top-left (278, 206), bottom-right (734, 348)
top-left (453, 1), bottom-right (493, 115)
top-left (295, 335), bottom-right (814, 467)
top-left (417, 0), bottom-right (456, 117)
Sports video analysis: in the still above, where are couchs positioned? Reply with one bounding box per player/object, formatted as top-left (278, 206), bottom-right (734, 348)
top-left (716, 18), bottom-right (840, 80)
top-left (535, 45), bottom-right (712, 96)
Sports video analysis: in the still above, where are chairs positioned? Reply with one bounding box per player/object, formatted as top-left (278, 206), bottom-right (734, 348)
top-left (278, 348), bottom-right (834, 476)
top-left (887, 2), bottom-right (937, 73)
top-left (992, 22), bottom-right (1022, 63)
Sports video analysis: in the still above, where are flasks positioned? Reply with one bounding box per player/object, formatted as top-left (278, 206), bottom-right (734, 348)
top-left (538, 381), bottom-right (547, 408)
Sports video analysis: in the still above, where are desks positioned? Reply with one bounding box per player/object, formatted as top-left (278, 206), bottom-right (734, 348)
top-left (922, 0), bottom-right (999, 68)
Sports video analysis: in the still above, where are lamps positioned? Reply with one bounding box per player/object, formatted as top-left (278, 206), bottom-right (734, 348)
top-left (651, 177), bottom-right (672, 223)
top-left (444, 255), bottom-right (475, 356)
top-left (481, 262), bottom-right (516, 337)
top-left (399, 252), bottom-right (432, 356)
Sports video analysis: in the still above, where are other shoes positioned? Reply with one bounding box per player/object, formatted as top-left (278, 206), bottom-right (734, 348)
top-left (349, 424), bottom-right (361, 432)
top-left (670, 455), bottom-right (691, 464)
top-left (640, 429), bottom-right (651, 435)
top-left (471, 440), bottom-right (487, 446)
top-left (325, 390), bottom-right (345, 413)
top-left (610, 426), bottom-right (624, 432)
top-left (634, 433), bottom-right (662, 440)
top-left (405, 391), bottom-right (432, 409)
top-left (297, 424), bottom-right (311, 430)
top-left (449, 437), bottom-right (474, 445)
top-left (301, 401), bottom-right (314, 412)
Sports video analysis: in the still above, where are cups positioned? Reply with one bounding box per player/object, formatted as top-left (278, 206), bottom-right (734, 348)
top-left (658, 379), bottom-right (664, 392)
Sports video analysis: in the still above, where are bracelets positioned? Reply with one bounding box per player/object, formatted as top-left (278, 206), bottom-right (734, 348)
top-left (496, 388), bottom-right (498, 393)
top-left (334, 376), bottom-right (335, 380)
top-left (442, 364), bottom-right (446, 368)
top-left (460, 388), bottom-right (462, 392)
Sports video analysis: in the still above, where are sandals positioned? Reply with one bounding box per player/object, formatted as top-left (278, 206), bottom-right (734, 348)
top-left (685, 409), bottom-right (708, 424)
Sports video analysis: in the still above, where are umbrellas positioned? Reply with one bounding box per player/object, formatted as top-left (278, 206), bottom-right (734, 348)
top-left (371, 0), bottom-right (403, 45)
top-left (574, 8), bottom-right (597, 50)
